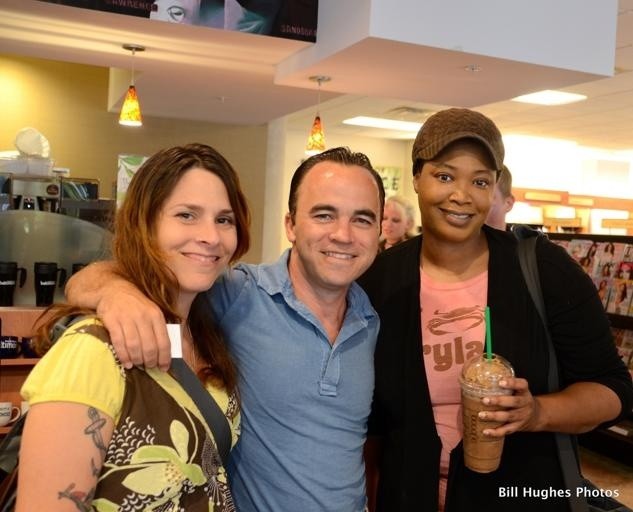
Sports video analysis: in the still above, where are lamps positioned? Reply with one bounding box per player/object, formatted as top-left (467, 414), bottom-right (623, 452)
top-left (117, 44), bottom-right (146, 127)
top-left (306, 76), bottom-right (332, 156)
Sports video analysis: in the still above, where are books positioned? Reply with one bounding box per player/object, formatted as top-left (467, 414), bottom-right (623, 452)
top-left (549, 240), bottom-right (632, 374)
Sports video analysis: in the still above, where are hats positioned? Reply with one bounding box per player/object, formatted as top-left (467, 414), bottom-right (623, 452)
top-left (412, 107), bottom-right (505, 183)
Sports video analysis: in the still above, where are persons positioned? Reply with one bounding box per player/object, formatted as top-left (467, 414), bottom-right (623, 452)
top-left (483, 161), bottom-right (516, 232)
top-left (377, 196), bottom-right (416, 251)
top-left (64, 149), bottom-right (388, 512)
top-left (12, 142), bottom-right (252, 512)
top-left (349, 107), bottom-right (633, 511)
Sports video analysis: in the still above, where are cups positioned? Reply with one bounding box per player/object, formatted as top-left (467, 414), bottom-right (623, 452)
top-left (34, 262), bottom-right (67, 307)
top-left (0, 335), bottom-right (23, 359)
top-left (457, 352), bottom-right (515, 474)
top-left (0, 402), bottom-right (21, 427)
top-left (0, 261), bottom-right (26, 307)
top-left (22, 336), bottom-right (41, 358)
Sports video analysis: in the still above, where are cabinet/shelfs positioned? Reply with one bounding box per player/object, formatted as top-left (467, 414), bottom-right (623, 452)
top-left (0, 169), bottom-right (118, 440)
top-left (546, 232), bottom-right (633, 473)
